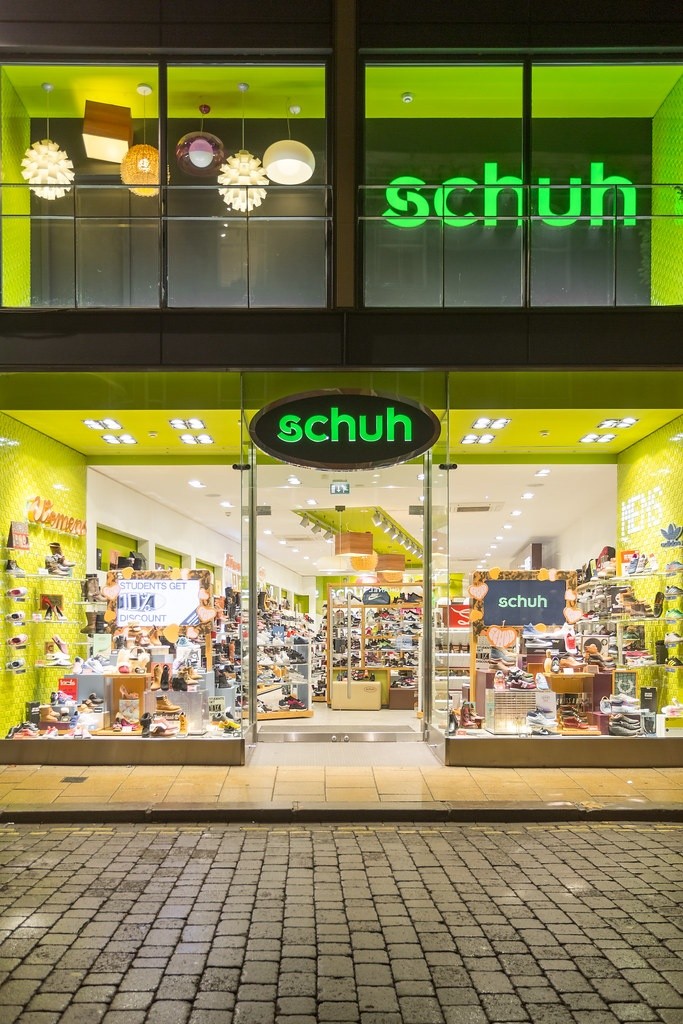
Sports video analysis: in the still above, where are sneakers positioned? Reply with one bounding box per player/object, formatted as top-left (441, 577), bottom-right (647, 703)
top-left (5, 537), bottom-right (683, 735)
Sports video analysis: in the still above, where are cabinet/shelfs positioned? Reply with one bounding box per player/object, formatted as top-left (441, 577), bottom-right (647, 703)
top-left (0, 542), bottom-right (683, 738)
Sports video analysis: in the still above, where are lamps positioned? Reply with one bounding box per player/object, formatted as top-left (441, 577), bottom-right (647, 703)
top-left (82, 100), bottom-right (133, 164)
top-left (409, 543), bottom-right (418, 555)
top-left (381, 518), bottom-right (390, 533)
top-left (119, 83), bottom-right (171, 197)
top-left (335, 532), bottom-right (373, 558)
top-left (403, 538), bottom-right (411, 550)
top-left (416, 548), bottom-right (422, 559)
top-left (351, 550), bottom-right (378, 571)
top-left (371, 511), bottom-right (382, 527)
top-left (397, 531), bottom-right (405, 545)
top-left (323, 530), bottom-right (333, 540)
top-left (299, 513), bottom-right (309, 528)
top-left (311, 523), bottom-right (321, 534)
top-left (375, 554), bottom-right (406, 572)
top-left (175, 105), bottom-right (229, 177)
top-left (217, 83), bottom-right (269, 212)
top-left (20, 84), bottom-right (75, 201)
top-left (262, 98), bottom-right (316, 186)
top-left (390, 525), bottom-right (398, 541)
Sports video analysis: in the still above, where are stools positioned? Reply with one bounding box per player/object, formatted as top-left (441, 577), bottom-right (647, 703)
top-left (388, 688), bottom-right (415, 710)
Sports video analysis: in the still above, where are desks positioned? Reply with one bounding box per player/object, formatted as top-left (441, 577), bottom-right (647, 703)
top-left (93, 672), bottom-right (154, 736)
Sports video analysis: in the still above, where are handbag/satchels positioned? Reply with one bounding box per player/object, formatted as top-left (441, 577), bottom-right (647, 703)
top-left (362, 586), bottom-right (390, 606)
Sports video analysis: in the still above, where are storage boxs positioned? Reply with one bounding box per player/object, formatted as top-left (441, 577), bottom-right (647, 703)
top-left (79, 711), bottom-right (110, 731)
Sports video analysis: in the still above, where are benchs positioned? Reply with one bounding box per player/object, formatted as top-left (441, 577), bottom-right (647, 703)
top-left (332, 681), bottom-right (380, 712)
